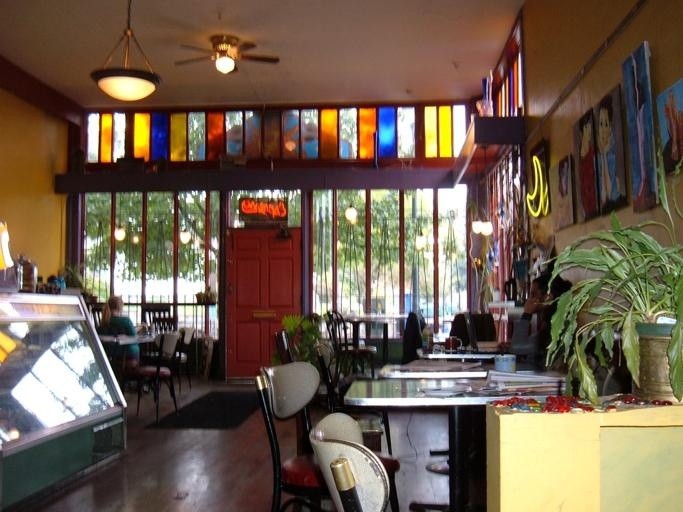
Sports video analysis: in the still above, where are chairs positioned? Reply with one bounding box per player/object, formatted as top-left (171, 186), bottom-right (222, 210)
top-left (255, 313), bottom-right (532, 512)
top-left (92, 302), bottom-right (194, 424)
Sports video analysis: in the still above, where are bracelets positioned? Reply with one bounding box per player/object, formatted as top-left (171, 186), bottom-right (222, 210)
top-left (520, 312), bottom-right (532, 321)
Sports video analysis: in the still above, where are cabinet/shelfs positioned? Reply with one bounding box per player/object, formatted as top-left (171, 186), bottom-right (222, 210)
top-left (0, 291), bottom-right (127, 512)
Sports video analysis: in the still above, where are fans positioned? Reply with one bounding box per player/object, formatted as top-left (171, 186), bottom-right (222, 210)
top-left (174, 12), bottom-right (280, 73)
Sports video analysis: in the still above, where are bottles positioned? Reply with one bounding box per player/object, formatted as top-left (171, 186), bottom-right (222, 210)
top-left (422, 323), bottom-right (433, 354)
top-left (36, 276), bottom-right (60, 294)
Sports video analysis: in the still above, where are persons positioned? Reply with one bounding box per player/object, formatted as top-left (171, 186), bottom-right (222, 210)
top-left (426, 272), bottom-right (576, 476)
top-left (576, 110), bottom-right (598, 222)
top-left (660, 90), bottom-right (683, 176)
top-left (97, 296), bottom-right (152, 394)
top-left (598, 89), bottom-right (628, 217)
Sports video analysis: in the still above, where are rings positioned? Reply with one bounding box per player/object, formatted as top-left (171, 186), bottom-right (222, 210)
top-left (534, 302), bottom-right (537, 304)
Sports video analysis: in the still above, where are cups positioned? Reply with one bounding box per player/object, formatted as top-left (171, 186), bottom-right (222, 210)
top-left (494, 354), bottom-right (516, 373)
top-left (445, 336), bottom-right (462, 353)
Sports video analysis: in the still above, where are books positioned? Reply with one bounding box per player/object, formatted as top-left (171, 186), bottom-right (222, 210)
top-left (485, 369), bottom-right (573, 395)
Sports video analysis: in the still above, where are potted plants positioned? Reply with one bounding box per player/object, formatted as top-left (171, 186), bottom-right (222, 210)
top-left (545, 144), bottom-right (683, 406)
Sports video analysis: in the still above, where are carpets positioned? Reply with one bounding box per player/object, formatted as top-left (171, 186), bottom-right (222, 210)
top-left (146, 391), bottom-right (260, 430)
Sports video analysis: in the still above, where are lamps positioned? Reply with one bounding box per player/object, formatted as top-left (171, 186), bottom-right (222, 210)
top-left (215, 57), bottom-right (235, 75)
top-left (89, 0), bottom-right (160, 101)
